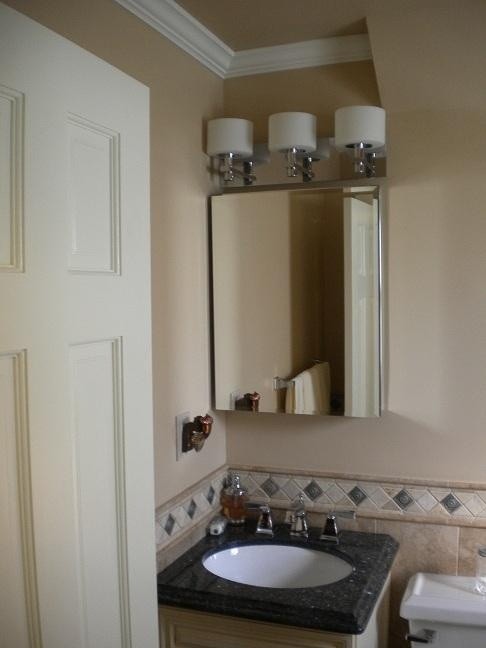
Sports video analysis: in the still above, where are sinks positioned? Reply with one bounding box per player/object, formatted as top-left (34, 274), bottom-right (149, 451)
top-left (200, 537), bottom-right (355, 589)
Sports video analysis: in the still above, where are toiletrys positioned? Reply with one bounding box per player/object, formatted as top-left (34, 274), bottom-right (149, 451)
top-left (221, 473), bottom-right (249, 524)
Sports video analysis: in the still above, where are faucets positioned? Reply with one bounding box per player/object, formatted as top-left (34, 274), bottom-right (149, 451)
top-left (286, 491), bottom-right (310, 537)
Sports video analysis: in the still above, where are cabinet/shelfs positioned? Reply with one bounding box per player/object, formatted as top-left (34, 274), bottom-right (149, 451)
top-left (157, 570), bottom-right (396, 647)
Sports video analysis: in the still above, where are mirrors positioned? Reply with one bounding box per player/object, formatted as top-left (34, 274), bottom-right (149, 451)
top-left (213, 184), bottom-right (380, 419)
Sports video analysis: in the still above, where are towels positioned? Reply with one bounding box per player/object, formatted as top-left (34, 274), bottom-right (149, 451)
top-left (283, 362), bottom-right (333, 417)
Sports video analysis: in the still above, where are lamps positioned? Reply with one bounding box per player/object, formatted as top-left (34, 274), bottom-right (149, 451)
top-left (199, 103), bottom-right (384, 184)
